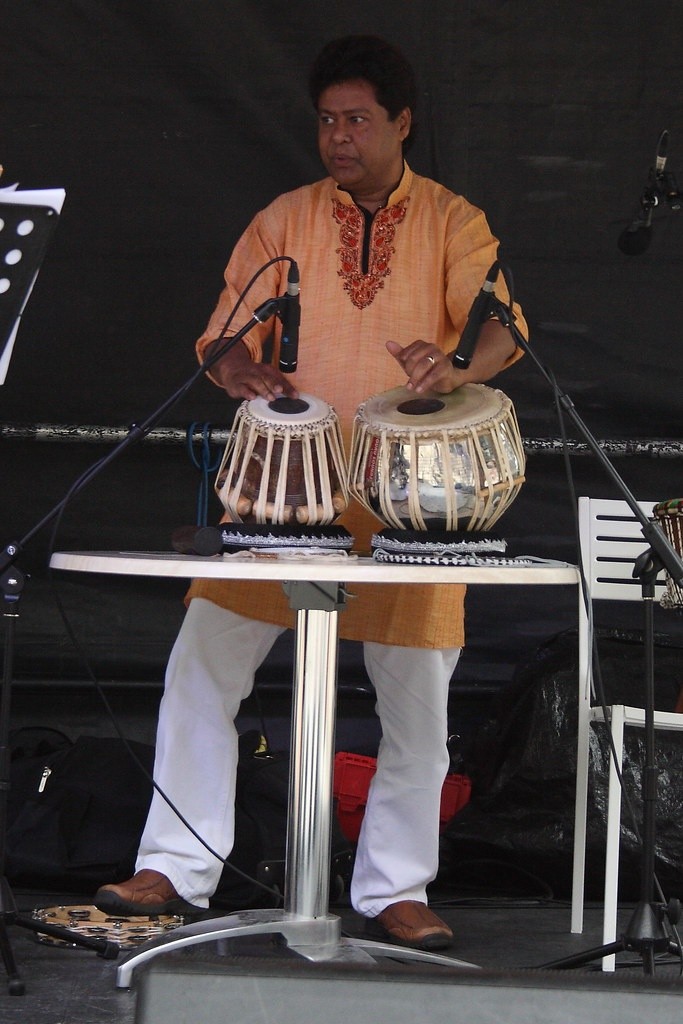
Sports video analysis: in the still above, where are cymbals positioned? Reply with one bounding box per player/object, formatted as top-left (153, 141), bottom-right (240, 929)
top-left (30, 904), bottom-right (185, 951)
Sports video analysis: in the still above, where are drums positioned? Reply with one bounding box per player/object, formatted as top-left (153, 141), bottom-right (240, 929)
top-left (346, 382), bottom-right (527, 534)
top-left (213, 387), bottom-right (351, 524)
top-left (652, 497), bottom-right (683, 608)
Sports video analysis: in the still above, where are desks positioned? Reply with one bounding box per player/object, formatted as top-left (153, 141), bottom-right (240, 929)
top-left (49, 550), bottom-right (584, 992)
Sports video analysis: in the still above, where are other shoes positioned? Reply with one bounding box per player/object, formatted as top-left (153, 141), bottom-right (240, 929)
top-left (95, 869), bottom-right (208, 917)
top-left (372, 900), bottom-right (454, 949)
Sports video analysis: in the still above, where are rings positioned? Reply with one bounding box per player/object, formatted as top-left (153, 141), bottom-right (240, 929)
top-left (426, 355), bottom-right (436, 364)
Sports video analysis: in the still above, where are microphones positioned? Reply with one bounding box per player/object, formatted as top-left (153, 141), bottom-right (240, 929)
top-left (451, 260), bottom-right (502, 370)
top-left (615, 130), bottom-right (670, 257)
top-left (278, 261), bottom-right (301, 374)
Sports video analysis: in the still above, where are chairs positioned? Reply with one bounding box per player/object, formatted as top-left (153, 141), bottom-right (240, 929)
top-left (572, 496), bottom-right (683, 972)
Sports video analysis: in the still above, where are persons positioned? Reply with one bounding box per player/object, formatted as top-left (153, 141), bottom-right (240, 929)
top-left (93, 36), bottom-right (528, 947)
